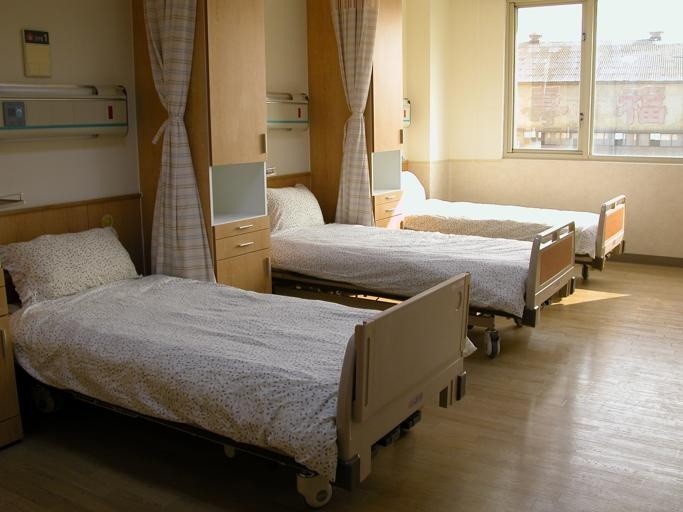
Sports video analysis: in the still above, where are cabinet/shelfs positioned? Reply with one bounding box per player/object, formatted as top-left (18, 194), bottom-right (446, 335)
top-left (132, 0), bottom-right (268, 294)
top-left (307, 0), bottom-right (404, 226)
